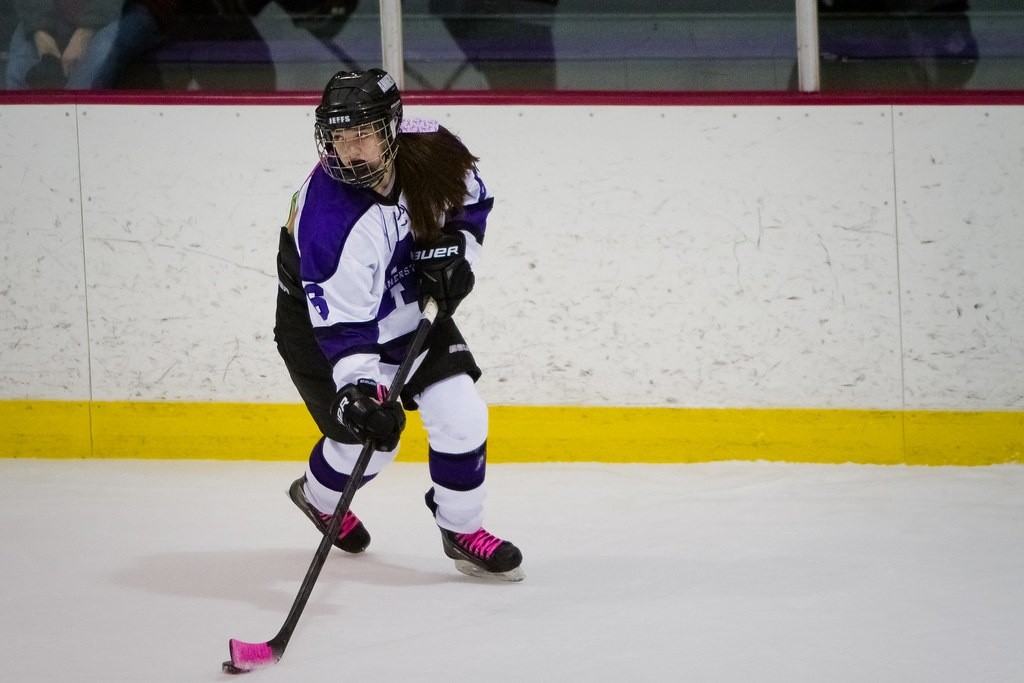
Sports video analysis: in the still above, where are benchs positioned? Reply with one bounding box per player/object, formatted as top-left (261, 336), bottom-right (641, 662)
top-left (120, 0), bottom-right (1024, 91)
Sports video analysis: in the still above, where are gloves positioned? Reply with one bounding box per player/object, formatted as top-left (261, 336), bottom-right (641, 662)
top-left (408, 227), bottom-right (475, 322)
top-left (330, 384), bottom-right (405, 451)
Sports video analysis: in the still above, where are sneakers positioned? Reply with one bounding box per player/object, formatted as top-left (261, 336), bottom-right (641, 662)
top-left (425, 487), bottom-right (526, 582)
top-left (289, 471), bottom-right (371, 553)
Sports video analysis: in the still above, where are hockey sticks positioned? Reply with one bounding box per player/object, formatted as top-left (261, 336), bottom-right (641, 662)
top-left (229, 300), bottom-right (437, 671)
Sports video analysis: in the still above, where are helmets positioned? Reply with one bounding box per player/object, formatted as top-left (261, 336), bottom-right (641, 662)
top-left (314, 68), bottom-right (402, 188)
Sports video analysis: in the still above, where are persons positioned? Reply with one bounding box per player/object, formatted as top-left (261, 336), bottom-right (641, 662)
top-left (272, 70), bottom-right (528, 582)
top-left (5, 0), bottom-right (144, 90)
